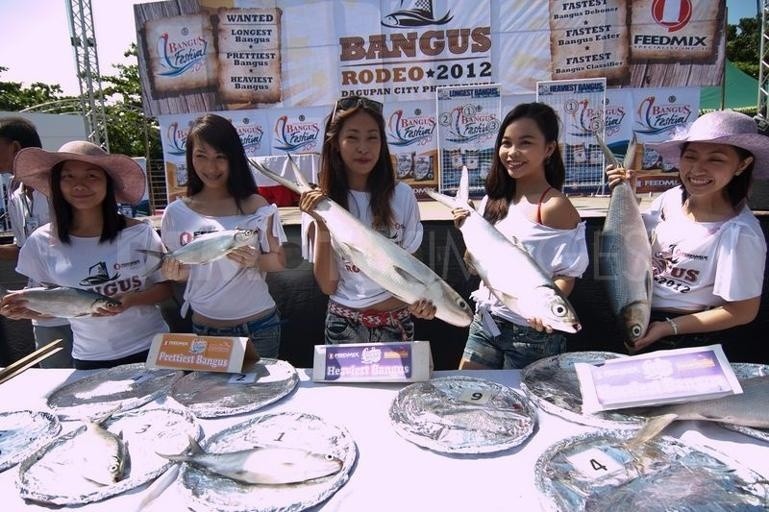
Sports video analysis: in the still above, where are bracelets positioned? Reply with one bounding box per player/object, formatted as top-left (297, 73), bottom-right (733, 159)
top-left (665, 317), bottom-right (678, 336)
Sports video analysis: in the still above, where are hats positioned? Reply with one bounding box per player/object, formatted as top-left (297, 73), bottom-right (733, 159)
top-left (13, 138), bottom-right (146, 210)
top-left (644, 112), bottom-right (769, 182)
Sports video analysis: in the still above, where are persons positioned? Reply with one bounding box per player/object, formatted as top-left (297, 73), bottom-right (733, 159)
top-left (0, 140), bottom-right (174, 372)
top-left (160, 114), bottom-right (288, 359)
top-left (605, 111), bottom-right (769, 362)
top-left (452, 103), bottom-right (590, 370)
top-left (0, 117), bottom-right (74, 368)
top-left (297, 96), bottom-right (436, 345)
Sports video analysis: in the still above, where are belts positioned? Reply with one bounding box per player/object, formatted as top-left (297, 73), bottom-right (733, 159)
top-left (474, 305), bottom-right (538, 335)
top-left (326, 299), bottom-right (411, 329)
top-left (191, 306), bottom-right (279, 336)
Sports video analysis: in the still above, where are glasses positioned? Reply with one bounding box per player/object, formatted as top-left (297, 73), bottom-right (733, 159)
top-left (328, 96), bottom-right (385, 127)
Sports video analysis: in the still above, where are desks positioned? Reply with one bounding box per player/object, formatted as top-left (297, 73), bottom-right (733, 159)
top-left (0, 360), bottom-right (769, 511)
top-left (0, 191), bottom-right (666, 283)
